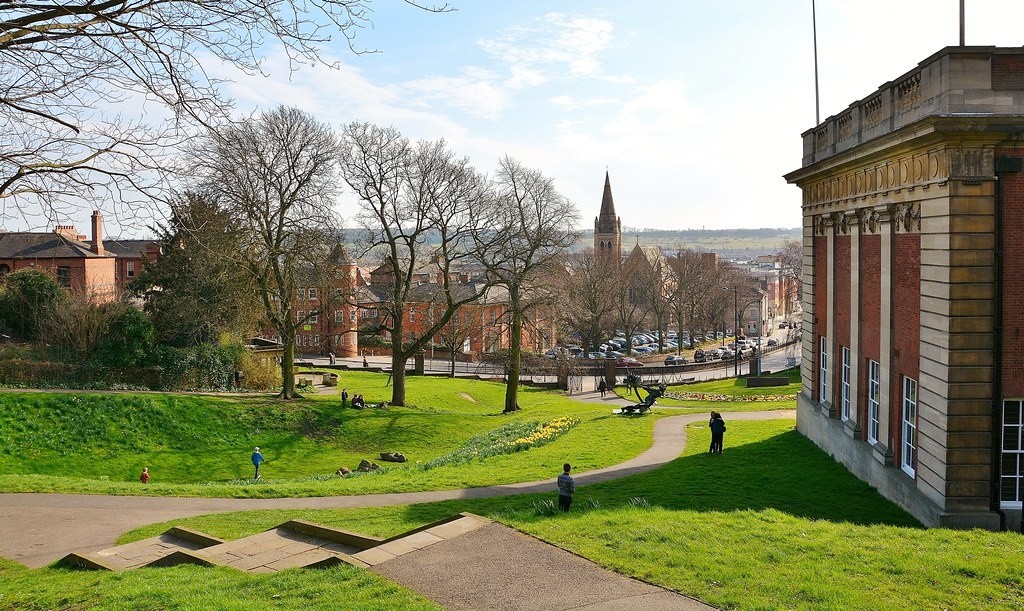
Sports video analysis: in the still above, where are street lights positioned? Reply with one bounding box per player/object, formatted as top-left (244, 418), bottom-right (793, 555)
top-left (723, 285), bottom-right (740, 376)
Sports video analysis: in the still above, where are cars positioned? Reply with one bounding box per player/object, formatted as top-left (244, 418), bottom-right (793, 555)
top-left (617, 357), bottom-right (643, 368)
top-left (779, 321), bottom-right (788, 330)
top-left (545, 326), bottom-right (764, 360)
top-left (664, 355), bottom-right (689, 366)
top-left (768, 338), bottom-right (779, 347)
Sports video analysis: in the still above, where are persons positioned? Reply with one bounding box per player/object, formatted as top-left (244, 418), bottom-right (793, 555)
top-left (251, 447), bottom-right (266, 479)
top-left (556, 463), bottom-right (575, 510)
top-left (709, 413), bottom-right (725, 454)
top-left (329, 353), bottom-right (335, 364)
top-left (341, 388), bottom-right (348, 406)
top-left (363, 355), bottom-right (369, 367)
top-left (598, 378), bottom-right (607, 398)
top-left (359, 395), bottom-right (365, 408)
top-left (709, 410), bottom-right (720, 452)
top-left (140, 467), bottom-right (150, 483)
top-left (352, 394), bottom-right (362, 409)
